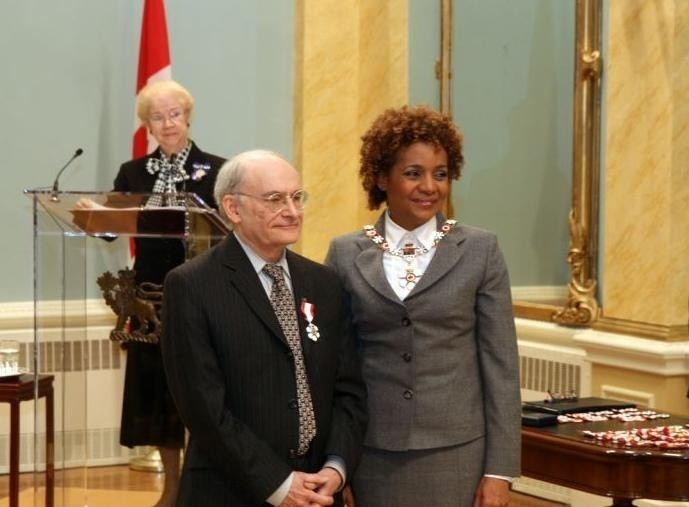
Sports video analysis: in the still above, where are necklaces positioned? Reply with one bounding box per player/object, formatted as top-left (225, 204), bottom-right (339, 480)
top-left (363, 218), bottom-right (458, 291)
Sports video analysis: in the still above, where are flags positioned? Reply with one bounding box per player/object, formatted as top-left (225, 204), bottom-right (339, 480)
top-left (123, 0), bottom-right (174, 260)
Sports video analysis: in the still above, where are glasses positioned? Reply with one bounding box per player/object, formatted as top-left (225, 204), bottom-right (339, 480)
top-left (233, 189), bottom-right (308, 213)
top-left (150, 110), bottom-right (181, 121)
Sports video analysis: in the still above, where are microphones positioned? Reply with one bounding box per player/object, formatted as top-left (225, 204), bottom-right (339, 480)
top-left (53, 148), bottom-right (83, 192)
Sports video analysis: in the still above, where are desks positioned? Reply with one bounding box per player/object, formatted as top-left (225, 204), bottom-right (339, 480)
top-left (22, 189), bottom-right (234, 503)
top-left (522, 406), bottom-right (686, 506)
top-left (3, 374), bottom-right (53, 506)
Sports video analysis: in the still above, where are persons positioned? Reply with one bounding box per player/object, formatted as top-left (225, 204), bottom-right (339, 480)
top-left (161, 150), bottom-right (369, 506)
top-left (323, 105), bottom-right (522, 507)
top-left (76, 80), bottom-right (228, 506)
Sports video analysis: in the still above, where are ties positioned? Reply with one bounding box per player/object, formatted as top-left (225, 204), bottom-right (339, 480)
top-left (263, 264), bottom-right (316, 456)
